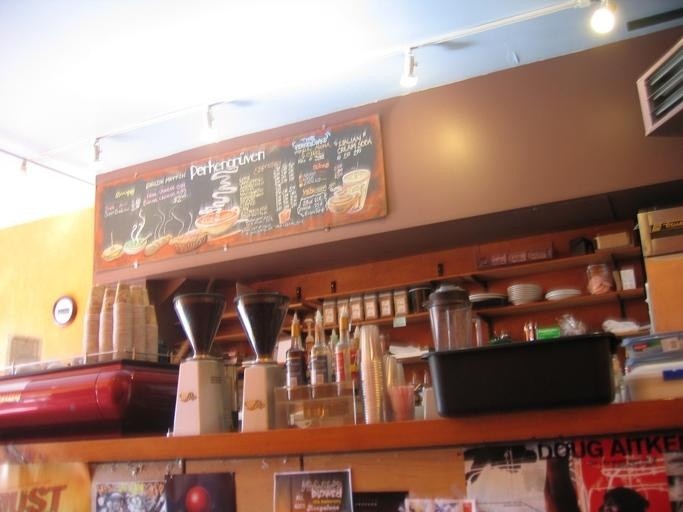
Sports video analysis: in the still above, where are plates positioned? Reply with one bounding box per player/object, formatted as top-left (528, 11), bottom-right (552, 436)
top-left (544, 288), bottom-right (581, 300)
top-left (468, 293), bottom-right (506, 305)
top-left (506, 284), bottom-right (541, 305)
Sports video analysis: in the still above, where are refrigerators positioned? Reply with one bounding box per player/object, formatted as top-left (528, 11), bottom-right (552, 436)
top-left (635, 206), bottom-right (683, 333)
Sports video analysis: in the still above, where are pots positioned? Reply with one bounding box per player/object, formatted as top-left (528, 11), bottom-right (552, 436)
top-left (194, 207), bottom-right (239, 236)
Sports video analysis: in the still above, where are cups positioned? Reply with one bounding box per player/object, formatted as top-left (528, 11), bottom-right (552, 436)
top-left (358, 324), bottom-right (385, 423)
top-left (383, 357), bottom-right (405, 385)
top-left (83, 282), bottom-right (159, 361)
top-left (342, 169), bottom-right (372, 214)
top-left (326, 192), bottom-right (360, 216)
top-left (388, 386), bottom-right (416, 420)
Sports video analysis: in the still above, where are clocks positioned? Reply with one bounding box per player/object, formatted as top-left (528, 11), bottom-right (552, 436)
top-left (52, 296), bottom-right (77, 327)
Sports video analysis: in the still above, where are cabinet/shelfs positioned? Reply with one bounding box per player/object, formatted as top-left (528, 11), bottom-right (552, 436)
top-left (153, 244), bottom-right (652, 364)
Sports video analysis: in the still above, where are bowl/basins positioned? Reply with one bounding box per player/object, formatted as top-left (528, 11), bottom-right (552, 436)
top-left (123, 238), bottom-right (147, 255)
top-left (100, 245), bottom-right (122, 262)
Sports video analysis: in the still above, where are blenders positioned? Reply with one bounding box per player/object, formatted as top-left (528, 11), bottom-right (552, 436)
top-left (421, 285), bottom-right (471, 418)
top-left (171, 292), bottom-right (228, 435)
top-left (232, 292), bottom-right (289, 433)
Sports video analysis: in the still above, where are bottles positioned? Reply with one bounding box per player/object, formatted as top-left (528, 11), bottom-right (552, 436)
top-left (310, 310), bottom-right (331, 384)
top-left (328, 328), bottom-right (337, 382)
top-left (305, 332), bottom-right (314, 384)
top-left (334, 307), bottom-right (355, 381)
top-left (352, 325), bottom-right (358, 380)
top-left (285, 311), bottom-right (306, 386)
